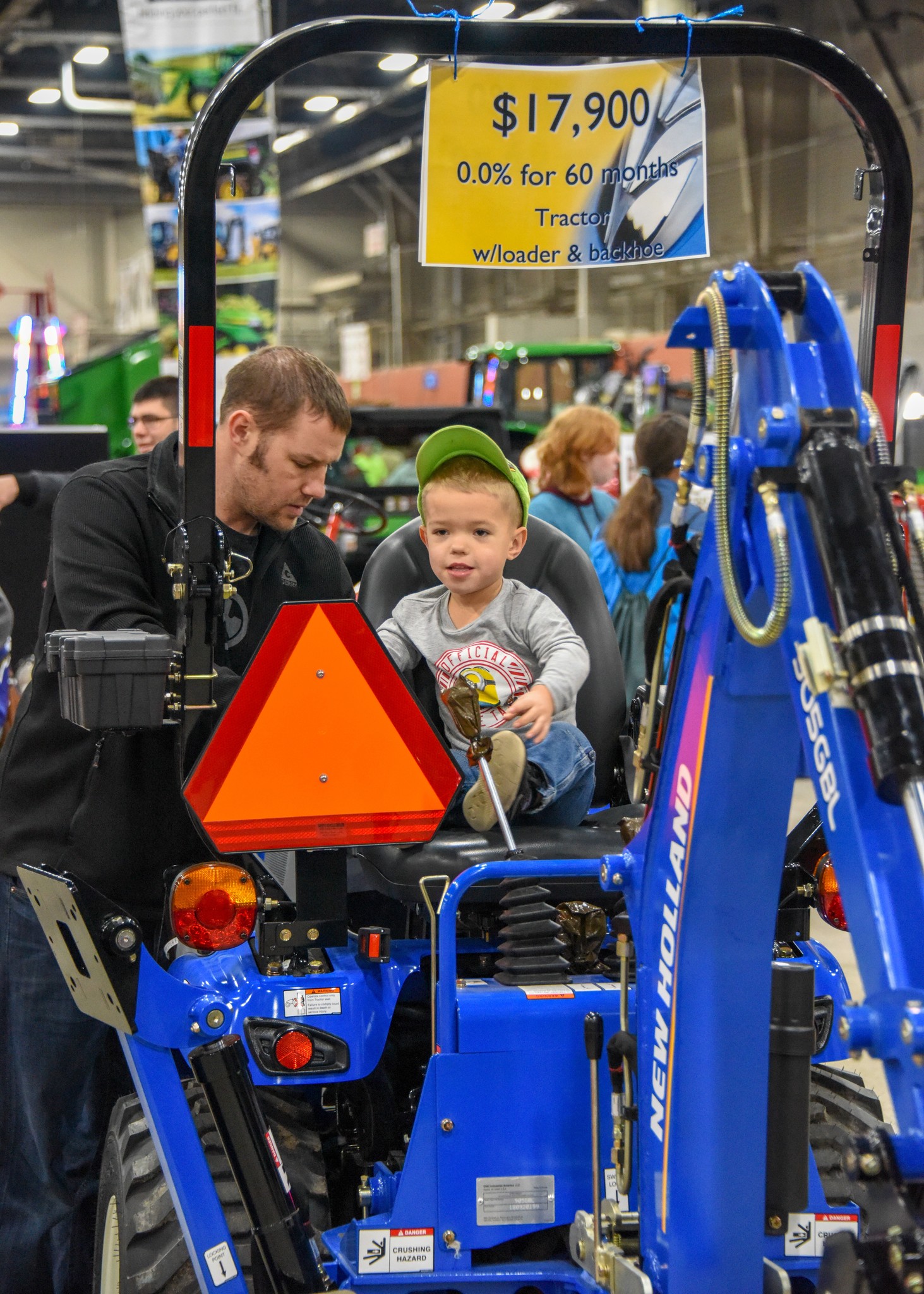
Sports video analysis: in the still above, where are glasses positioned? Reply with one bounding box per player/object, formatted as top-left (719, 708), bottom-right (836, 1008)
top-left (125, 416), bottom-right (178, 428)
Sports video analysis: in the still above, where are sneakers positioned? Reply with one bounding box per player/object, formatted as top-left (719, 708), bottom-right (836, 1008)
top-left (463, 729), bottom-right (527, 833)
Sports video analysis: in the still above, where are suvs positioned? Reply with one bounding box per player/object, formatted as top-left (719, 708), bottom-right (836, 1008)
top-left (89, 14), bottom-right (924, 1293)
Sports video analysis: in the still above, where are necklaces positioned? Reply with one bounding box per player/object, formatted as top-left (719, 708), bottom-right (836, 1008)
top-left (571, 493), bottom-right (603, 538)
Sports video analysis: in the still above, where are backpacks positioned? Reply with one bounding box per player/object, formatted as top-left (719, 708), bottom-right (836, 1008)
top-left (607, 510), bottom-right (705, 729)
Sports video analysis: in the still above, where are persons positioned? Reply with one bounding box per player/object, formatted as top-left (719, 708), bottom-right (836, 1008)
top-left (373, 424), bottom-right (597, 853)
top-left (0, 375), bottom-right (179, 513)
top-left (528, 403), bottom-right (623, 558)
top-left (0, 344), bottom-right (358, 1294)
top-left (586, 412), bottom-right (707, 736)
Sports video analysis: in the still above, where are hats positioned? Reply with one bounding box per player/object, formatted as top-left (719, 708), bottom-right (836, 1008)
top-left (415, 424), bottom-right (530, 529)
